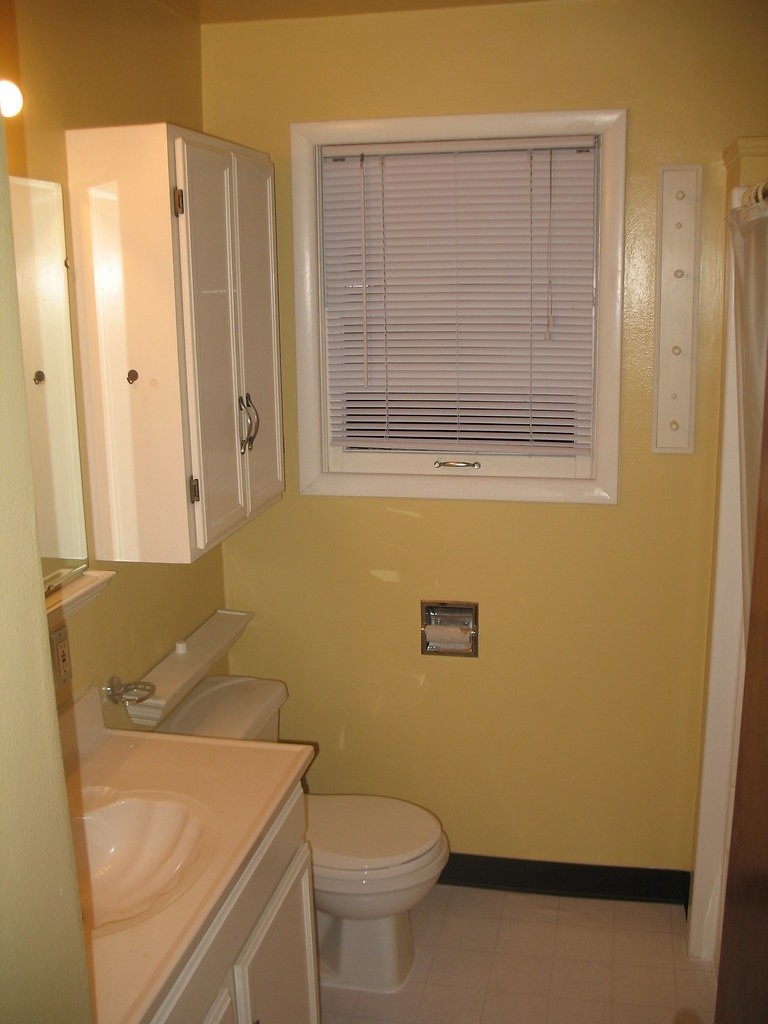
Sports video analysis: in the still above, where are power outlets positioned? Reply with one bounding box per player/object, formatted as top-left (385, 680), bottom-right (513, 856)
top-left (49, 626), bottom-right (73, 687)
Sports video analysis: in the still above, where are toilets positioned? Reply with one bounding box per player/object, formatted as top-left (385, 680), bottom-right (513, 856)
top-left (148, 669), bottom-right (450, 997)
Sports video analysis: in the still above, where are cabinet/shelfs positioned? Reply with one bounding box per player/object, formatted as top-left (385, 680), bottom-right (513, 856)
top-left (3, 176), bottom-right (88, 560)
top-left (148, 781), bottom-right (322, 1024)
top-left (64, 123), bottom-right (286, 566)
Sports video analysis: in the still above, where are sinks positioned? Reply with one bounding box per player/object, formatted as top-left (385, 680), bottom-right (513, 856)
top-left (68, 784), bottom-right (208, 944)
